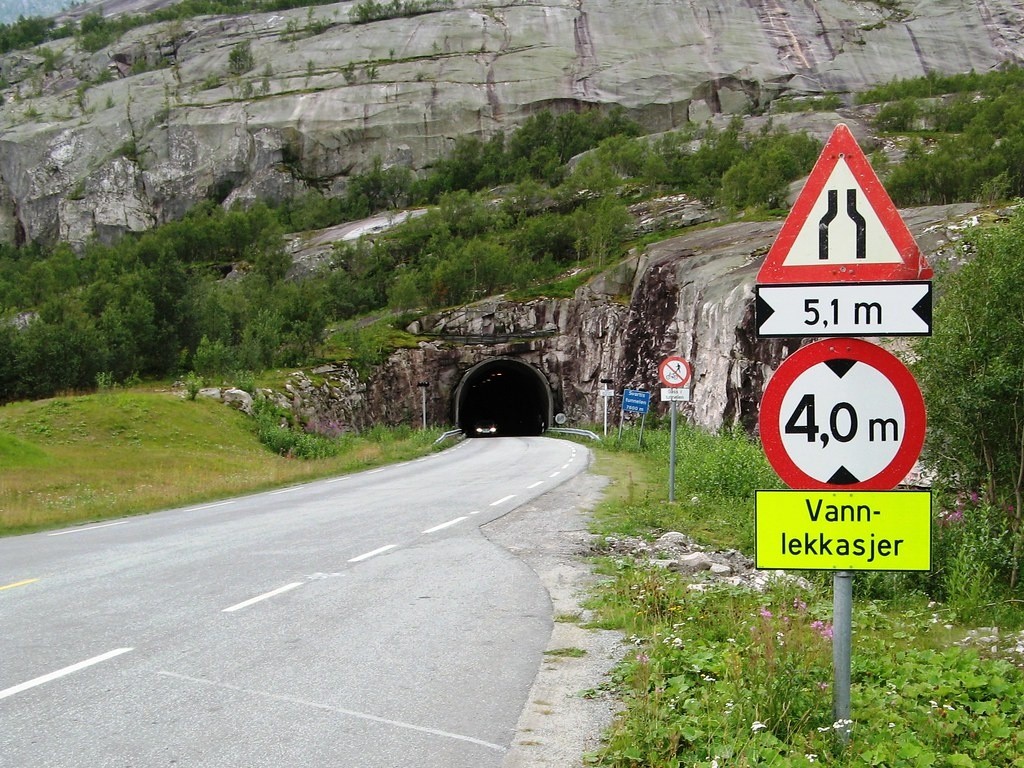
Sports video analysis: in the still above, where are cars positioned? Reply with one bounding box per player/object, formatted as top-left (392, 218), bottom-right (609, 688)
top-left (473, 419), bottom-right (498, 437)
